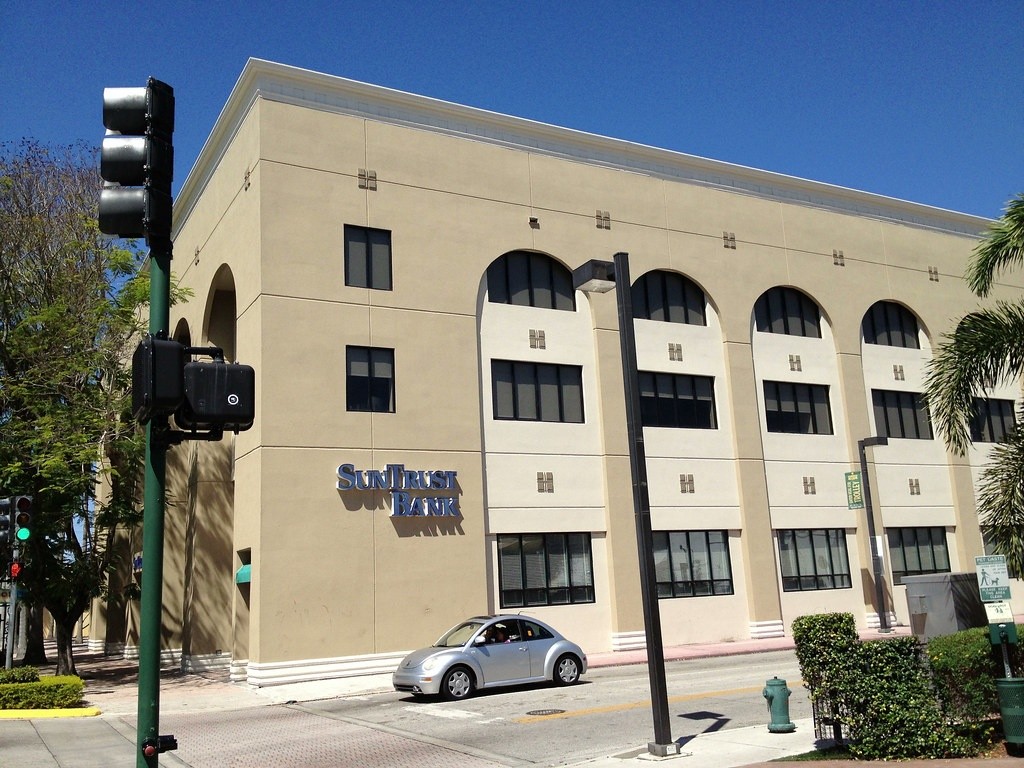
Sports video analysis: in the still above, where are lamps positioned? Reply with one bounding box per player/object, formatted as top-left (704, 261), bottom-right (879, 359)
top-left (529, 217), bottom-right (537, 223)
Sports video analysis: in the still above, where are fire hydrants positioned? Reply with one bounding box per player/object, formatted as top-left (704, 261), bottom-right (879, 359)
top-left (761, 676), bottom-right (796, 734)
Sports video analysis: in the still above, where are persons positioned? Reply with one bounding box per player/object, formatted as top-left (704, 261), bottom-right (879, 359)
top-left (496, 627), bottom-right (511, 644)
top-left (486, 624), bottom-right (498, 643)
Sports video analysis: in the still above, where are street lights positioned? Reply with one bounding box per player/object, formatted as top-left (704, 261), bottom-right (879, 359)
top-left (854, 435), bottom-right (894, 633)
top-left (570, 251), bottom-right (681, 757)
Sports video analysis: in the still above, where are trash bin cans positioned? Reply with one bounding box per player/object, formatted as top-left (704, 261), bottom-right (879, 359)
top-left (994, 677), bottom-right (1024, 742)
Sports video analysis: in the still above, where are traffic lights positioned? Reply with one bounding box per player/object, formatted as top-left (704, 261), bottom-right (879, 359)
top-left (129, 329), bottom-right (192, 425)
top-left (92, 70), bottom-right (177, 247)
top-left (0, 495), bottom-right (14, 549)
top-left (15, 495), bottom-right (32, 548)
top-left (8, 561), bottom-right (25, 579)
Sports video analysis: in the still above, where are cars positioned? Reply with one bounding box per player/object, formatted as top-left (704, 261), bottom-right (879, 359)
top-left (390, 612), bottom-right (588, 701)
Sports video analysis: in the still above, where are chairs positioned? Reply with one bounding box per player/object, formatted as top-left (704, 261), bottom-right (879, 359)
top-left (539, 628), bottom-right (547, 636)
top-left (526, 626), bottom-right (536, 639)
